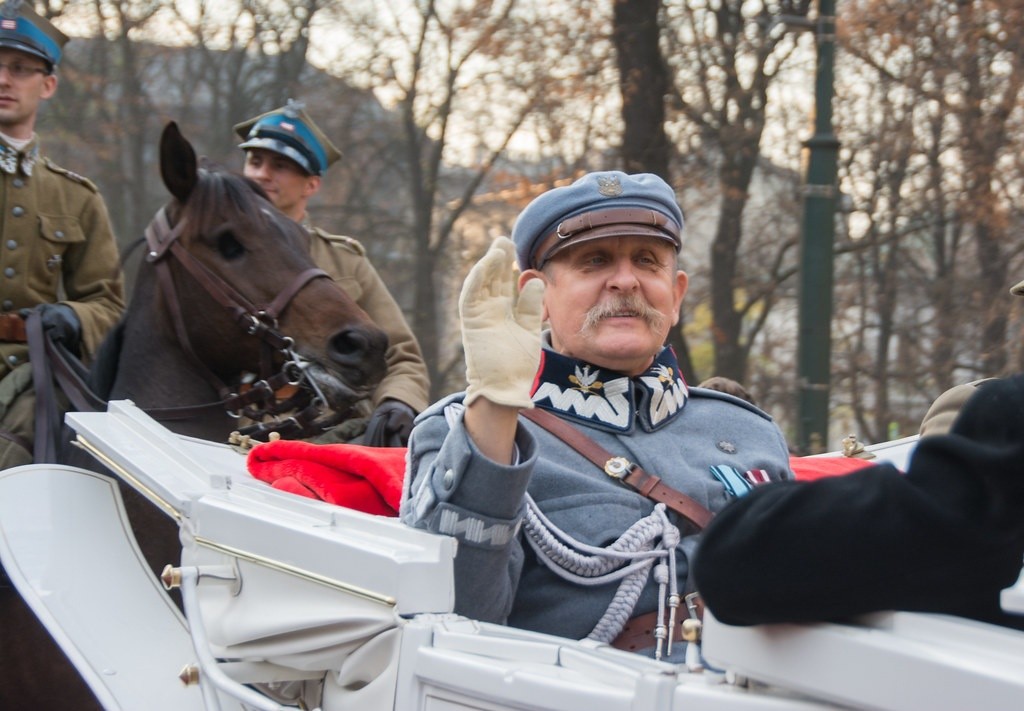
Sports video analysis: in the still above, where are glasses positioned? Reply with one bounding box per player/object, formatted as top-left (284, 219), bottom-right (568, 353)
top-left (2, 63), bottom-right (52, 79)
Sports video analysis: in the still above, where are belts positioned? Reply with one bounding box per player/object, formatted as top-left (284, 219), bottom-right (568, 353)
top-left (0, 314), bottom-right (30, 342)
top-left (614, 591), bottom-right (703, 649)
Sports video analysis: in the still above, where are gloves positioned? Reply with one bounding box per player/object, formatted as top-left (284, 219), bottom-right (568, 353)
top-left (373, 398), bottom-right (415, 440)
top-left (20, 304), bottom-right (82, 354)
top-left (458, 237), bottom-right (544, 411)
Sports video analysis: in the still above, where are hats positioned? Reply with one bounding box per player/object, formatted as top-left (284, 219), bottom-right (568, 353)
top-left (0, 0), bottom-right (72, 69)
top-left (508, 170), bottom-right (683, 270)
top-left (232, 98), bottom-right (342, 178)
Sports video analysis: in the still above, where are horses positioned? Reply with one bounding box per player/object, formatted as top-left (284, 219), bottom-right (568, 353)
top-left (0, 119), bottom-right (391, 711)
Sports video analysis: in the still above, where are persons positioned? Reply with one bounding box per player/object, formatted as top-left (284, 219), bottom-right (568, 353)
top-left (0, 1), bottom-right (127, 465)
top-left (231, 99), bottom-right (430, 448)
top-left (917, 277), bottom-right (1023, 434)
top-left (690, 366), bottom-right (1022, 633)
top-left (397, 171), bottom-right (793, 674)
